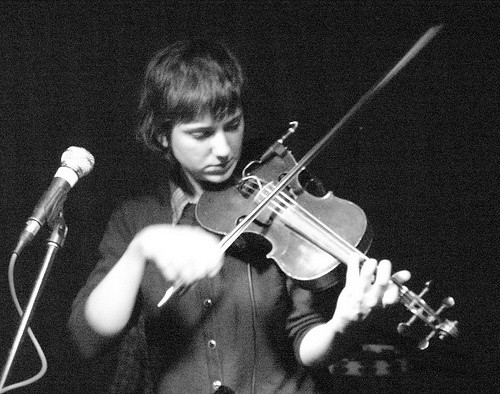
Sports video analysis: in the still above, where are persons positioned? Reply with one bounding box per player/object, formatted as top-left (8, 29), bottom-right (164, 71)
top-left (70, 36), bottom-right (411, 394)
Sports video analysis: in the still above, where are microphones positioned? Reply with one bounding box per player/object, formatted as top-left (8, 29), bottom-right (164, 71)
top-left (261, 126), bottom-right (296, 163)
top-left (13, 145), bottom-right (96, 255)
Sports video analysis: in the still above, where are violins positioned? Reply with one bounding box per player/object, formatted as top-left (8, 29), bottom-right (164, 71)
top-left (194, 121), bottom-right (460, 350)
